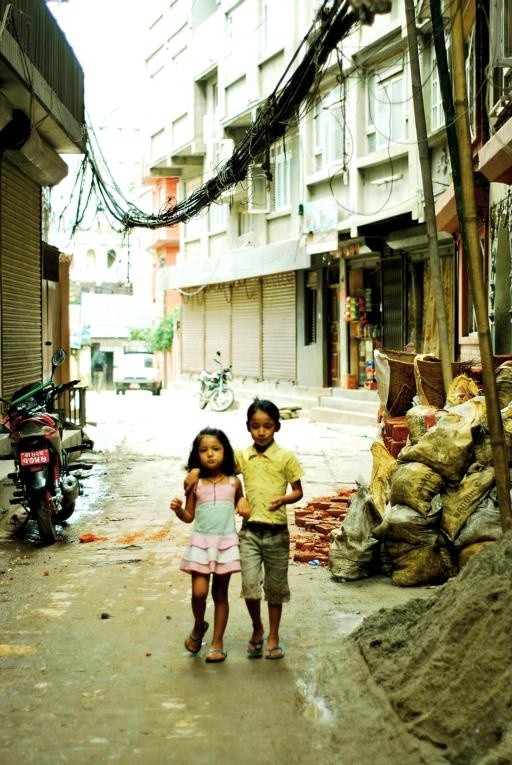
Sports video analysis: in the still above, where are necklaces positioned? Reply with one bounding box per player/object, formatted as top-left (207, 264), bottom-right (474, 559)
top-left (205, 473), bottom-right (224, 505)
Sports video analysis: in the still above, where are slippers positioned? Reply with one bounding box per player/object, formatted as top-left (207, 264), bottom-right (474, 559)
top-left (246, 637), bottom-right (265, 657)
top-left (264, 644), bottom-right (285, 660)
top-left (204, 648), bottom-right (227, 663)
top-left (183, 620), bottom-right (210, 654)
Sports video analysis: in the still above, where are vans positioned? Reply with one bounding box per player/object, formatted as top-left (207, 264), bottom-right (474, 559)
top-left (112, 344), bottom-right (164, 395)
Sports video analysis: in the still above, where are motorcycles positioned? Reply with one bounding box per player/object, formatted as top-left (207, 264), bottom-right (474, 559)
top-left (197, 351), bottom-right (234, 412)
top-left (0, 349), bottom-right (94, 546)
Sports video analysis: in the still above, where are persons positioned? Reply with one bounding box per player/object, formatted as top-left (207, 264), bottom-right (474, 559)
top-left (93, 352), bottom-right (105, 392)
top-left (184, 400), bottom-right (303, 659)
top-left (171, 427), bottom-right (251, 662)
